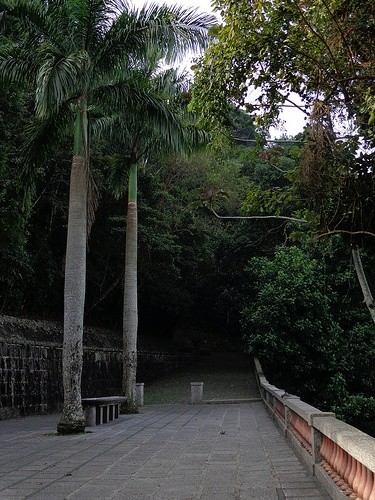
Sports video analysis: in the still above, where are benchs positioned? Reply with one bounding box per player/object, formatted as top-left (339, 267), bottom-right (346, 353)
top-left (82, 396), bottom-right (129, 428)
